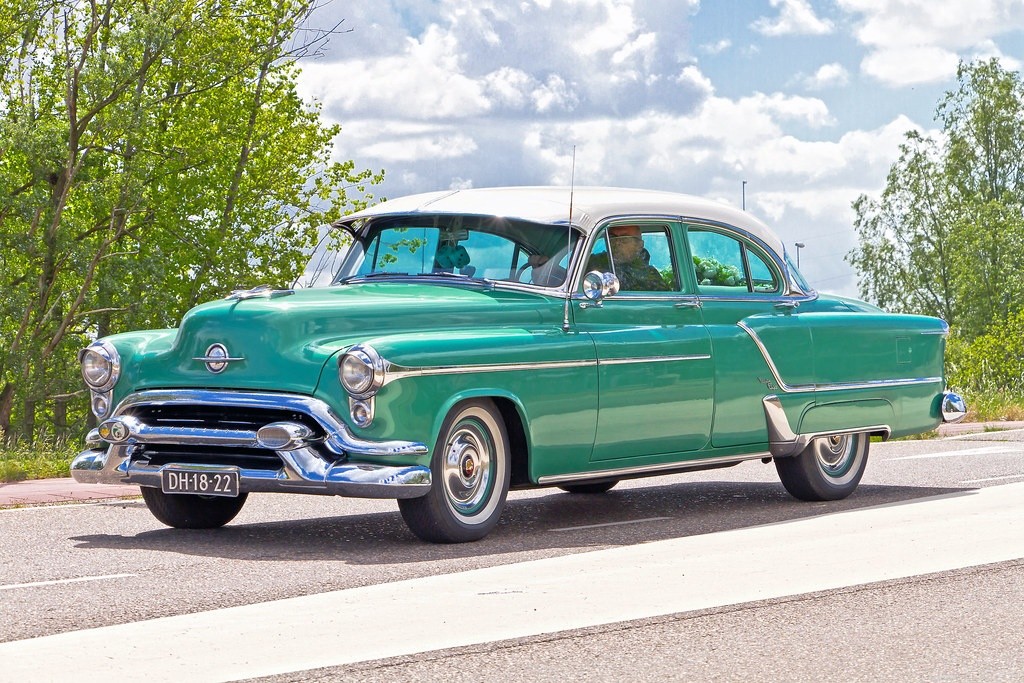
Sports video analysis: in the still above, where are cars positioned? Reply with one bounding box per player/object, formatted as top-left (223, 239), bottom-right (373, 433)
top-left (69, 185), bottom-right (967, 545)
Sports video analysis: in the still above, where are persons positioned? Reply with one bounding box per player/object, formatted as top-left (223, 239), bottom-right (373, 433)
top-left (528, 225), bottom-right (664, 291)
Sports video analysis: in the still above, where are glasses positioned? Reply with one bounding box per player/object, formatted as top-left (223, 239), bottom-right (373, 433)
top-left (606, 235), bottom-right (641, 246)
